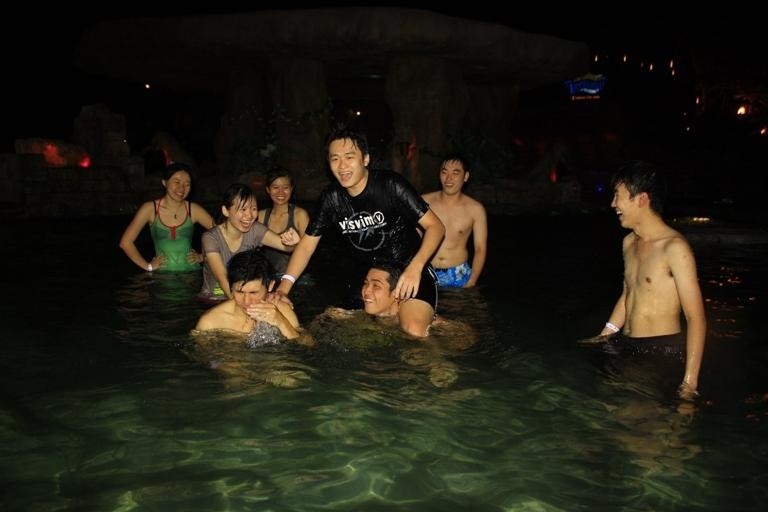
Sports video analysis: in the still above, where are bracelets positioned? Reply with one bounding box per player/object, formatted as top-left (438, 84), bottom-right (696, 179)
top-left (280, 275), bottom-right (296, 283)
top-left (603, 320), bottom-right (620, 333)
top-left (148, 261), bottom-right (156, 270)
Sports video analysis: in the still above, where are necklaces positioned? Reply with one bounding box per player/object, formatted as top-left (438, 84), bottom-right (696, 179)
top-left (164, 203), bottom-right (185, 221)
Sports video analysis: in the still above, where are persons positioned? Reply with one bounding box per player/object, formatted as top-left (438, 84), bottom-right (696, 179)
top-left (598, 354), bottom-right (702, 481)
top-left (312, 260), bottom-right (478, 372)
top-left (118, 160), bottom-right (219, 277)
top-left (256, 168), bottom-right (318, 290)
top-left (180, 240), bottom-right (319, 398)
top-left (264, 125), bottom-right (462, 390)
top-left (200, 183), bottom-right (301, 307)
top-left (414, 149), bottom-right (490, 290)
top-left (578, 157), bottom-right (703, 401)
